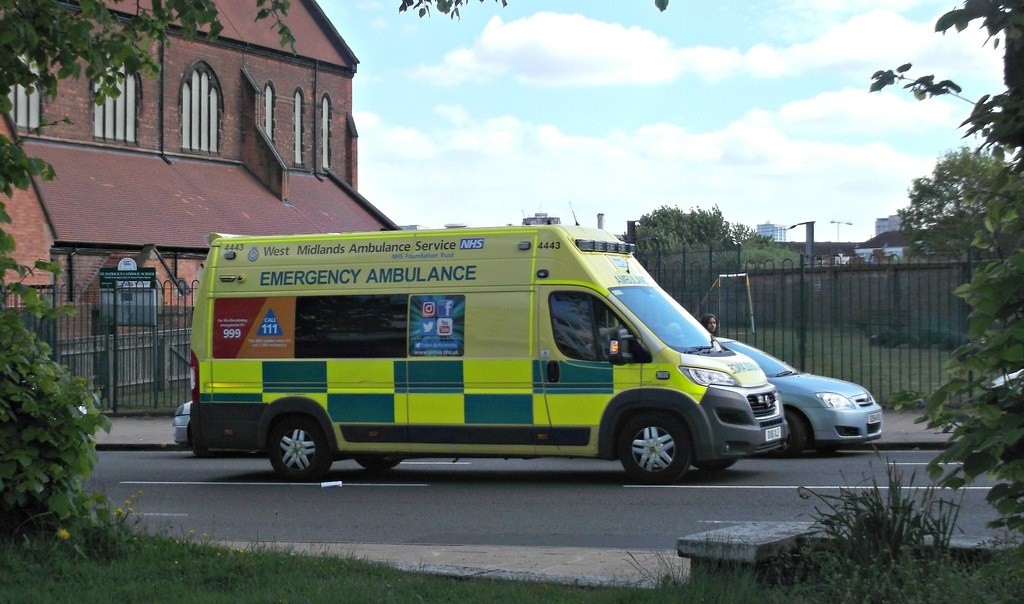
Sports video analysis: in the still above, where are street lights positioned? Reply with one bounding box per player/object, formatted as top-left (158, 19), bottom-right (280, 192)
top-left (830, 220), bottom-right (853, 242)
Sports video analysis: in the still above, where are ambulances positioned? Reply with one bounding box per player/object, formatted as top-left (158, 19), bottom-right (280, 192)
top-left (188, 212), bottom-right (790, 489)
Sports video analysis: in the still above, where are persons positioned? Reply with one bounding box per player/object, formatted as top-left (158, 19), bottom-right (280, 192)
top-left (565, 298), bottom-right (606, 361)
top-left (701, 314), bottom-right (717, 336)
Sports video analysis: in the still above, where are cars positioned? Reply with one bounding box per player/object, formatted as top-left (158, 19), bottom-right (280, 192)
top-left (714, 336), bottom-right (885, 459)
